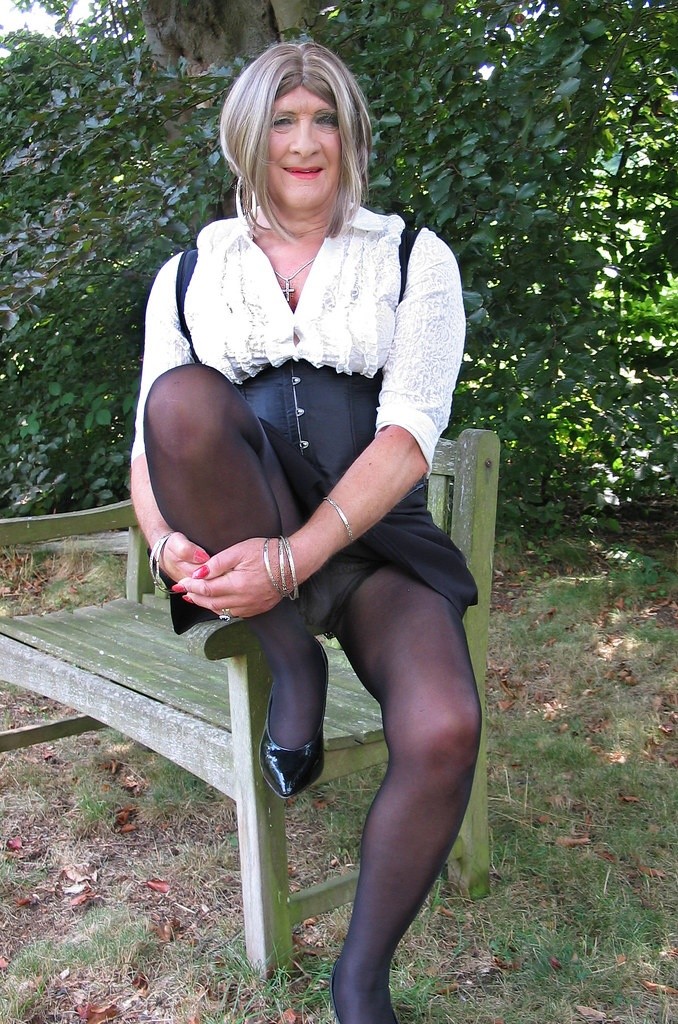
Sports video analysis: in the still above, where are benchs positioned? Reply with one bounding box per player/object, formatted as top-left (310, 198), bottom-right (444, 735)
top-left (0, 427), bottom-right (503, 986)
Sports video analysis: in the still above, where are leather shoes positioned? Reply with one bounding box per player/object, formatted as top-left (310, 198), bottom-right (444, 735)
top-left (259, 638), bottom-right (330, 798)
top-left (330, 958), bottom-right (398, 1024)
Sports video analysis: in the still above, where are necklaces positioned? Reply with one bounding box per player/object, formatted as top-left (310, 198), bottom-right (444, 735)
top-left (275, 259), bottom-right (314, 301)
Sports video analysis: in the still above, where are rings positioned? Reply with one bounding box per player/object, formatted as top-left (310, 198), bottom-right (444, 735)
top-left (220, 608), bottom-right (233, 622)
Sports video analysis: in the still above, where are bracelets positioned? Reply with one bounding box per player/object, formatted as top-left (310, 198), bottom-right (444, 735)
top-left (149, 535), bottom-right (179, 594)
top-left (264, 535), bottom-right (299, 601)
top-left (323, 497), bottom-right (354, 542)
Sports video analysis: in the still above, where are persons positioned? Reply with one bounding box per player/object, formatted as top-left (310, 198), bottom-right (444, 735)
top-left (129, 40), bottom-right (483, 1023)
